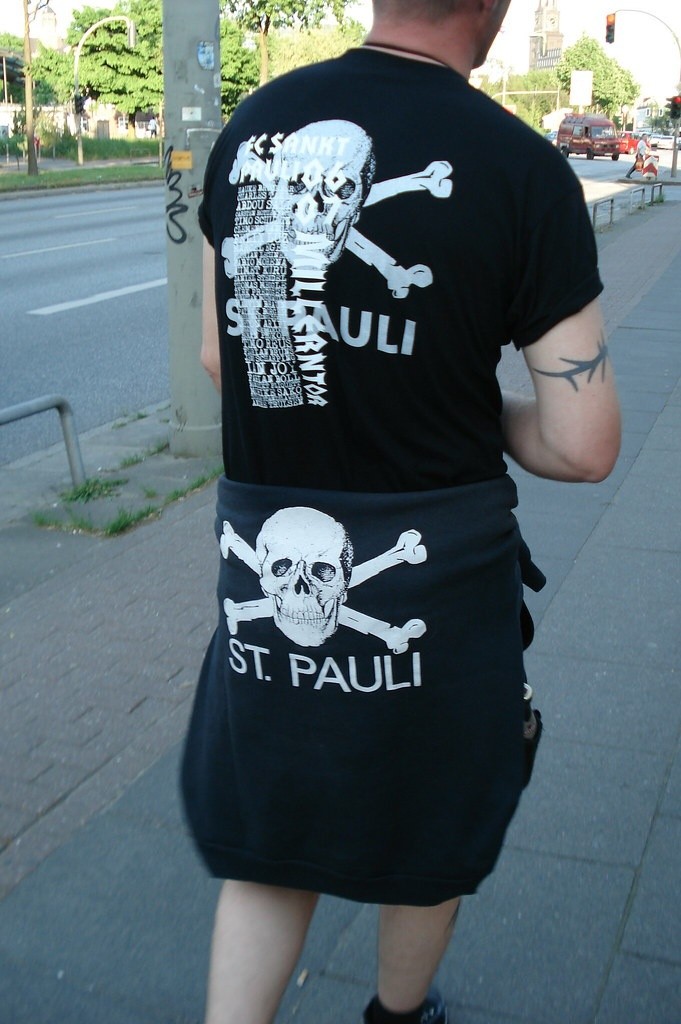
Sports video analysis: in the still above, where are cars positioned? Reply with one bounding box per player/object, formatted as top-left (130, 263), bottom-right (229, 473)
top-left (617, 129), bottom-right (640, 153)
top-left (650, 135), bottom-right (661, 145)
top-left (677, 138), bottom-right (681, 149)
top-left (658, 136), bottom-right (674, 150)
top-left (544, 133), bottom-right (558, 146)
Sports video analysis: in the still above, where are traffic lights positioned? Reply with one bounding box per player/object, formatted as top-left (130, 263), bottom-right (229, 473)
top-left (605, 12), bottom-right (615, 42)
top-left (75, 94), bottom-right (84, 114)
top-left (666, 97), bottom-right (673, 116)
top-left (670, 96), bottom-right (681, 121)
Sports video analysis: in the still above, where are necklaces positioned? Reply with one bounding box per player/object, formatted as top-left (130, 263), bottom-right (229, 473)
top-left (362, 42), bottom-right (446, 66)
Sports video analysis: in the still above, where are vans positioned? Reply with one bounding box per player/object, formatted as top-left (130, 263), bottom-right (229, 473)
top-left (557, 114), bottom-right (621, 161)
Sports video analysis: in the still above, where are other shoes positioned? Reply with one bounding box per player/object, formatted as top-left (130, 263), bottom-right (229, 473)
top-left (363, 987), bottom-right (447, 1024)
top-left (626, 175), bottom-right (632, 179)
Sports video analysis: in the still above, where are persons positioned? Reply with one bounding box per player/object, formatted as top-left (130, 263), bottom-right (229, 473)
top-left (150, 117), bottom-right (156, 138)
top-left (180, 1), bottom-right (621, 1023)
top-left (626, 134), bottom-right (651, 179)
top-left (602, 128), bottom-right (609, 136)
